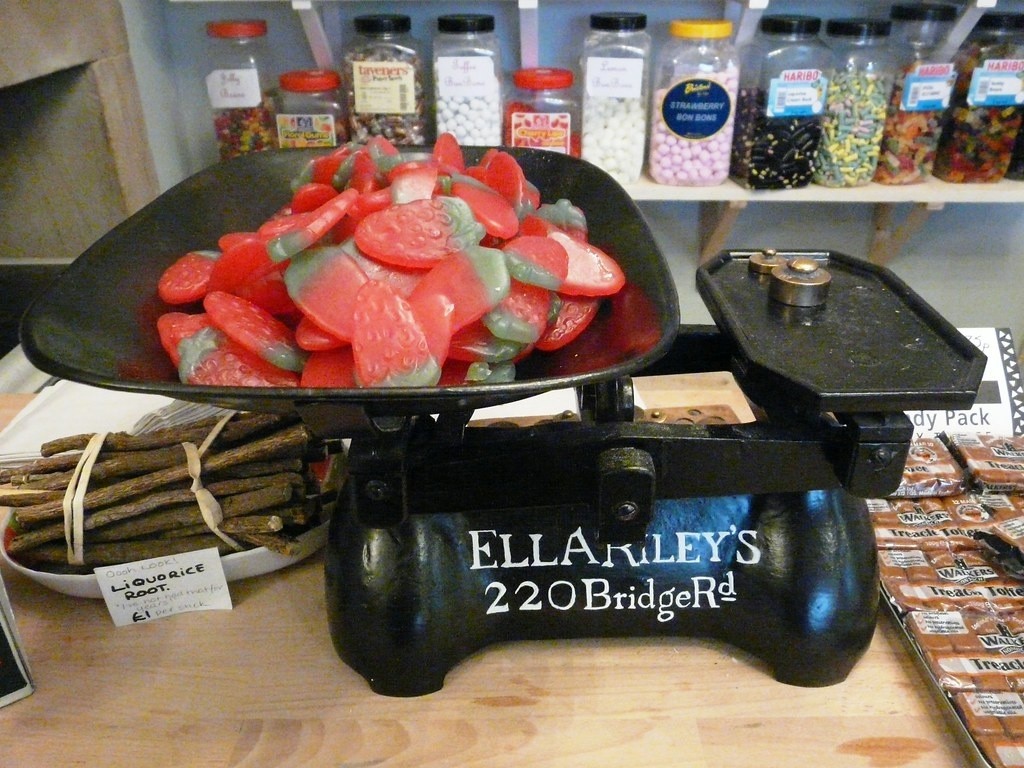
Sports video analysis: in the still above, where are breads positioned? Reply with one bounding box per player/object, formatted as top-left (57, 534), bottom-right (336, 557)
top-left (869, 433), bottom-right (1024, 768)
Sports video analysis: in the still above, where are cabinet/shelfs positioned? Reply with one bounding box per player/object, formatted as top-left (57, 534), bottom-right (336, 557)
top-left (167, 0), bottom-right (1023, 336)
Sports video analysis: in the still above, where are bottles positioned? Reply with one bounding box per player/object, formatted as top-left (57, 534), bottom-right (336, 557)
top-left (340, 13), bottom-right (430, 148)
top-left (866, 3), bottom-right (962, 186)
top-left (578, 11), bottom-right (652, 187)
top-left (811, 17), bottom-right (898, 190)
top-left (430, 14), bottom-right (505, 147)
top-left (729, 13), bottom-right (835, 191)
top-left (202, 18), bottom-right (279, 159)
top-left (501, 66), bottom-right (583, 159)
top-left (644, 18), bottom-right (742, 187)
top-left (933, 9), bottom-right (1024, 187)
top-left (275, 68), bottom-right (348, 151)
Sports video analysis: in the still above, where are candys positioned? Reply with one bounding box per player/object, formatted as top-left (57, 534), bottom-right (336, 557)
top-left (875, 54), bottom-right (1024, 184)
top-left (157, 135), bottom-right (628, 386)
top-left (212, 46), bottom-right (424, 161)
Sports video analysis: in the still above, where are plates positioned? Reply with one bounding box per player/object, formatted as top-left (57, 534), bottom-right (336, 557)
top-left (1, 508), bottom-right (332, 599)
